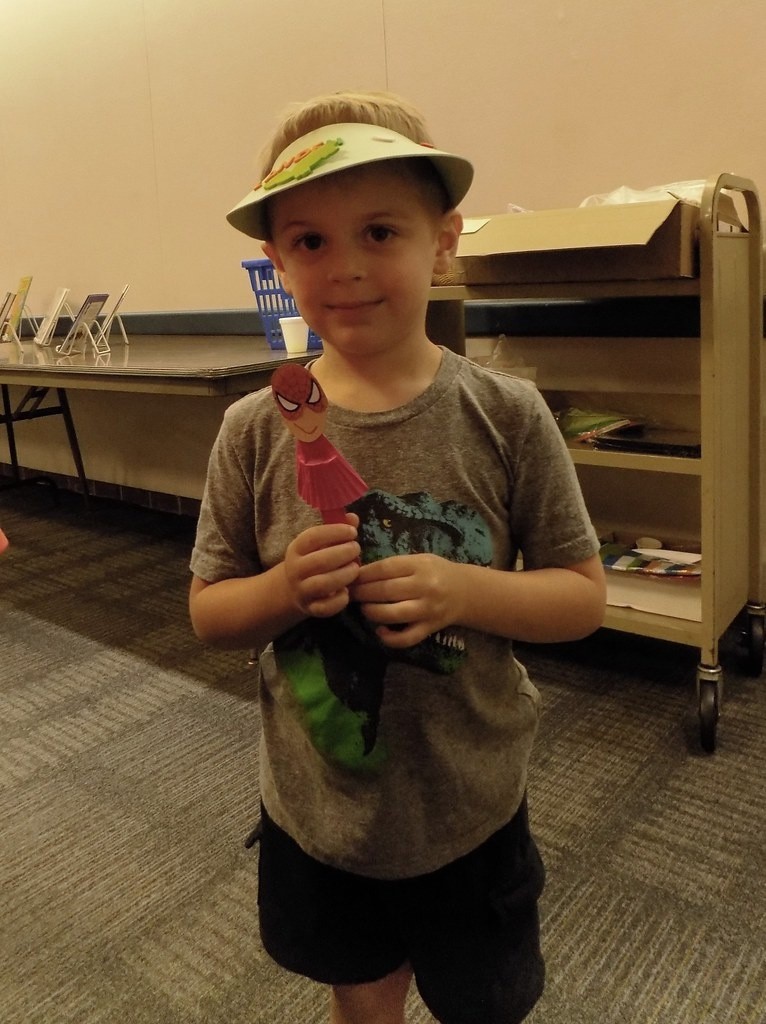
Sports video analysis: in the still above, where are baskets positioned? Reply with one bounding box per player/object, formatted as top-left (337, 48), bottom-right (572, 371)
top-left (242, 259), bottom-right (323, 350)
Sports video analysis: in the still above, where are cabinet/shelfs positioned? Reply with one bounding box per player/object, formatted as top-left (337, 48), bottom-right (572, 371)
top-left (456, 176), bottom-right (766, 758)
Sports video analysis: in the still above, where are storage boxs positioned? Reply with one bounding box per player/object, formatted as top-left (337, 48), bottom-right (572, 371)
top-left (242, 258), bottom-right (323, 351)
top-left (452, 188), bottom-right (701, 289)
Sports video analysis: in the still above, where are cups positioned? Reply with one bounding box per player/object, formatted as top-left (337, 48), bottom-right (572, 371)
top-left (278, 316), bottom-right (309, 354)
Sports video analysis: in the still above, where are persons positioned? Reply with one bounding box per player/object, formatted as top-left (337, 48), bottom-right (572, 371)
top-left (191, 91), bottom-right (607, 1024)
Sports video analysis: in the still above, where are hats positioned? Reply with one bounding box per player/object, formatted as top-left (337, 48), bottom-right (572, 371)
top-left (226, 122), bottom-right (474, 240)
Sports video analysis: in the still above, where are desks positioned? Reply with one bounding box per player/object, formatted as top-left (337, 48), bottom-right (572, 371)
top-left (0, 332), bottom-right (327, 516)
